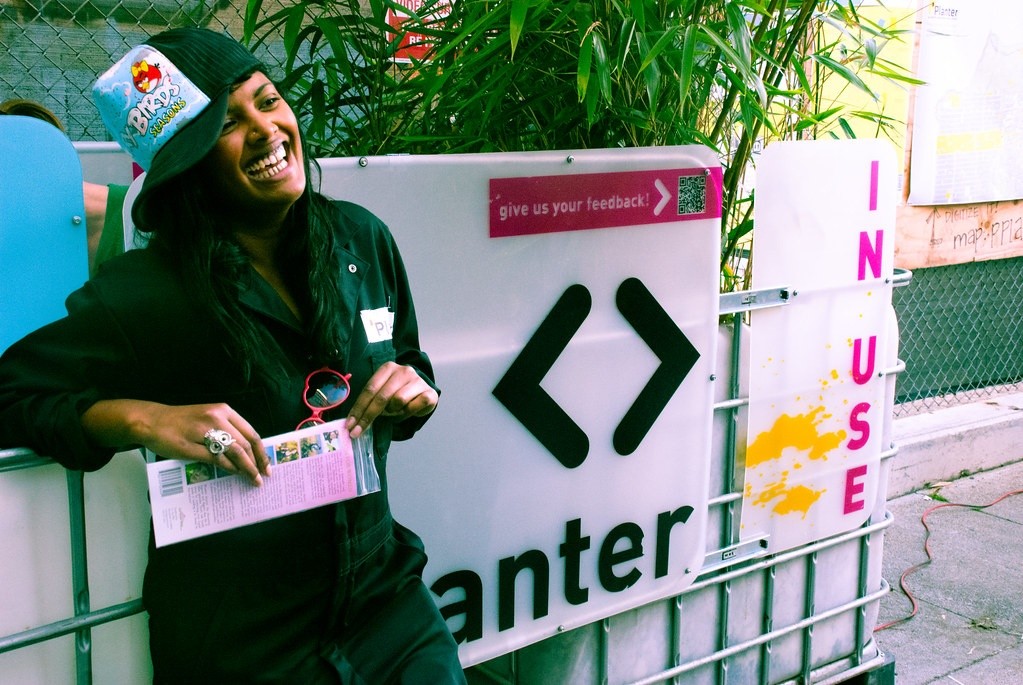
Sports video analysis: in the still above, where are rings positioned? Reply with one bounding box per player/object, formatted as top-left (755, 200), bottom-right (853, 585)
top-left (203, 428), bottom-right (237, 455)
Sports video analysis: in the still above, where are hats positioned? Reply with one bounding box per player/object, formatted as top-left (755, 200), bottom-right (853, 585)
top-left (91, 27), bottom-right (266, 232)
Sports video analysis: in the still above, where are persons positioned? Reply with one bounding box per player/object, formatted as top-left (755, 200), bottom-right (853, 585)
top-left (0, 99), bottom-right (137, 282)
top-left (0, 27), bottom-right (469, 685)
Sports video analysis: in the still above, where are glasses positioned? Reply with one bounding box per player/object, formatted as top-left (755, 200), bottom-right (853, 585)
top-left (295, 366), bottom-right (352, 431)
